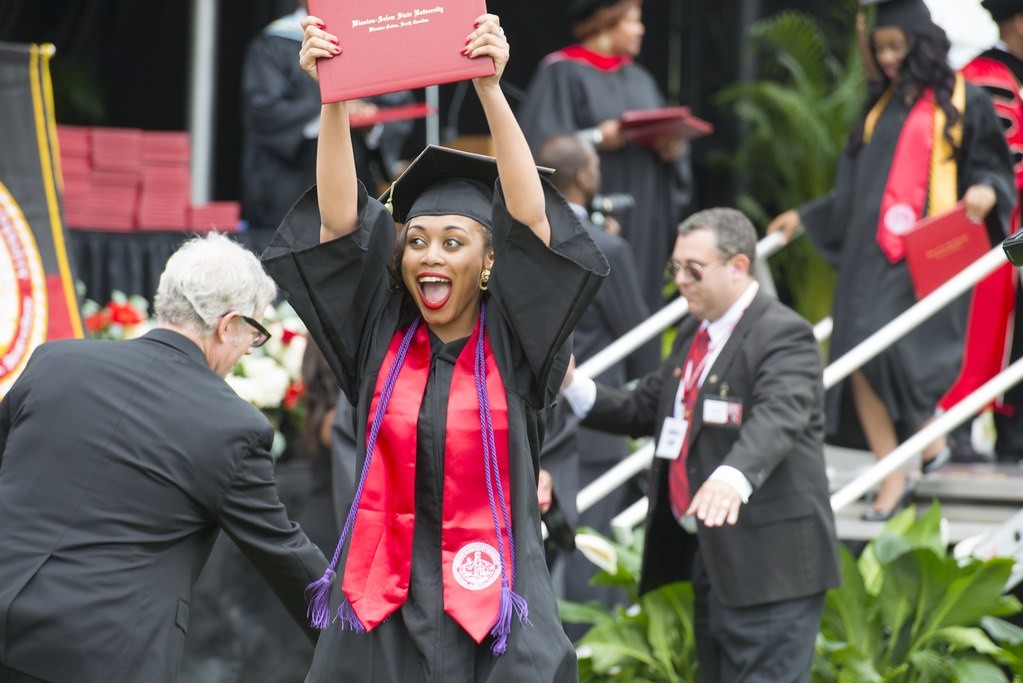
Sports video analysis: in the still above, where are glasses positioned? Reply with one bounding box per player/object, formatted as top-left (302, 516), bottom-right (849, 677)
top-left (222, 310), bottom-right (272, 347)
top-left (666, 251), bottom-right (753, 283)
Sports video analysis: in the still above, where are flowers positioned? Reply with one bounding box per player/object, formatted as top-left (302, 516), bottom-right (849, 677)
top-left (75, 281), bottom-right (308, 419)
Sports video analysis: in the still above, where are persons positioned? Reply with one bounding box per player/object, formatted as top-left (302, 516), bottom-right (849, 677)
top-left (259, 14), bottom-right (608, 683)
top-left (960, 1), bottom-right (1023, 466)
top-left (768, 0), bottom-right (1017, 522)
top-left (538, 141), bottom-right (655, 641)
top-left (243, 0), bottom-right (414, 236)
top-left (0, 234), bottom-right (338, 683)
top-left (298, 333), bottom-right (581, 572)
top-left (563, 207), bottom-right (840, 683)
top-left (522, 1), bottom-right (693, 335)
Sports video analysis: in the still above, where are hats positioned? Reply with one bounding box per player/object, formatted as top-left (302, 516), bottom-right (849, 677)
top-left (378, 144), bottom-right (556, 232)
top-left (855, 0), bottom-right (931, 82)
top-left (568, 0), bottom-right (621, 21)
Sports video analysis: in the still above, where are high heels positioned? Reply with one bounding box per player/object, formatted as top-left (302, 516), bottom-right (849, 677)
top-left (923, 447), bottom-right (953, 476)
top-left (859, 471), bottom-right (921, 521)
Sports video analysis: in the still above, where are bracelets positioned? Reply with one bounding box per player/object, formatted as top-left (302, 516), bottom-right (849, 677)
top-left (592, 126), bottom-right (603, 147)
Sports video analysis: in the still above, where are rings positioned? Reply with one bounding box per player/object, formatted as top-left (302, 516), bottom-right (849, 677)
top-left (723, 500), bottom-right (732, 506)
top-left (498, 27), bottom-right (504, 37)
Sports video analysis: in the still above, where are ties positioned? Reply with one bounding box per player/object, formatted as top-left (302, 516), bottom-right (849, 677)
top-left (668, 319), bottom-right (711, 522)
